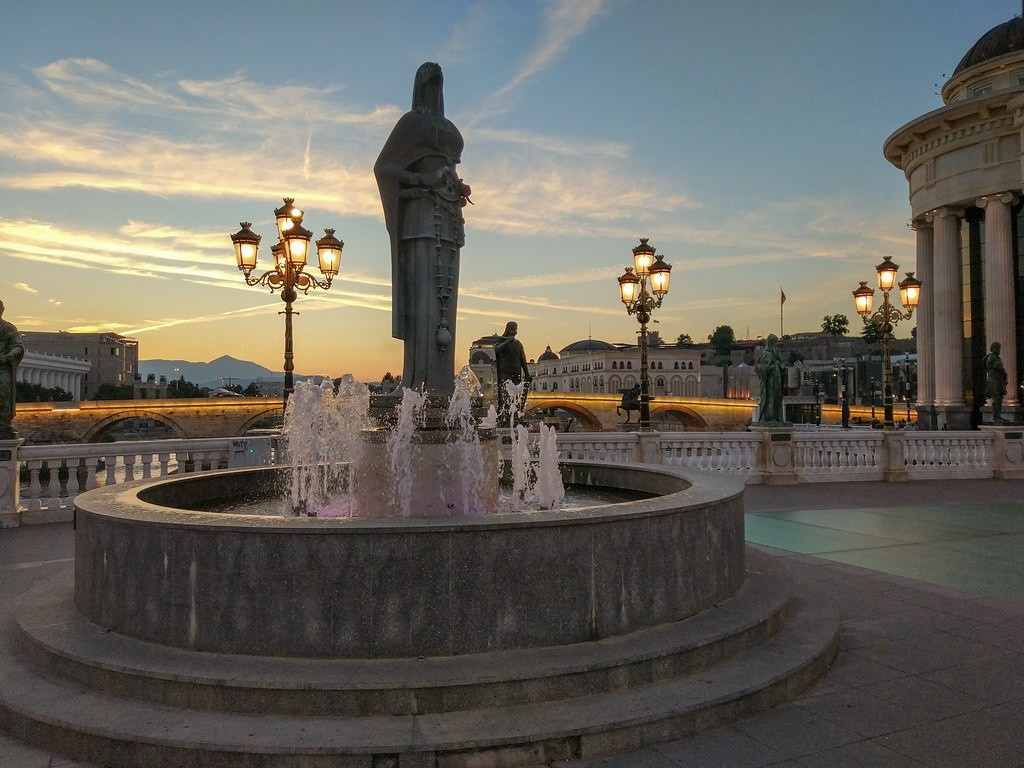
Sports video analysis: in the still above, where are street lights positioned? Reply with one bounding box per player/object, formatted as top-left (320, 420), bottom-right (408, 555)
top-left (231, 196), bottom-right (346, 432)
top-left (850, 254), bottom-right (923, 432)
top-left (616, 237), bottom-right (672, 431)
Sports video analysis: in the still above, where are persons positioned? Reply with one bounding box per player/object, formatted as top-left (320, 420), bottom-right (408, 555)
top-left (896, 376), bottom-right (905, 402)
top-left (373, 61), bottom-right (471, 394)
top-left (632, 383), bottom-right (641, 404)
top-left (494, 322), bottom-right (533, 427)
top-left (983, 342), bottom-right (1010, 421)
top-left (0, 301), bottom-right (24, 432)
top-left (754, 334), bottom-right (787, 422)
